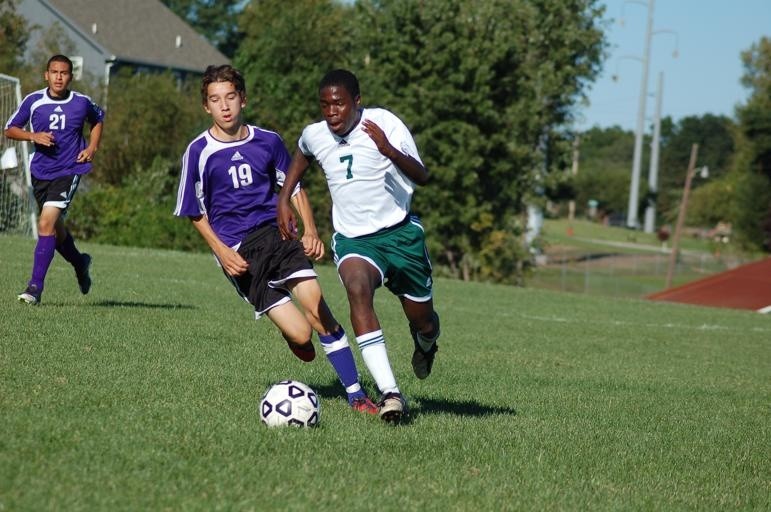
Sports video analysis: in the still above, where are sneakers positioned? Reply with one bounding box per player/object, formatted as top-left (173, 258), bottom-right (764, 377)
top-left (282, 330), bottom-right (318, 363)
top-left (379, 390), bottom-right (410, 424)
top-left (16, 285), bottom-right (45, 307)
top-left (74, 252), bottom-right (93, 296)
top-left (345, 382), bottom-right (378, 415)
top-left (408, 320), bottom-right (441, 381)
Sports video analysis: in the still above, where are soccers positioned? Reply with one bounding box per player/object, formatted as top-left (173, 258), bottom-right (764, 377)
top-left (260, 380), bottom-right (319, 429)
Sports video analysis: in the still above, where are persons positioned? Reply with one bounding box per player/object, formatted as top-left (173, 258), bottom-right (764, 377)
top-left (278, 69), bottom-right (440, 420)
top-left (174, 64), bottom-right (378, 415)
top-left (3, 54), bottom-right (107, 304)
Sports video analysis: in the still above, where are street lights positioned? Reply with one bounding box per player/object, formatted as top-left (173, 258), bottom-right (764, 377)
top-left (610, 1), bottom-right (684, 229)
top-left (661, 164), bottom-right (714, 292)
